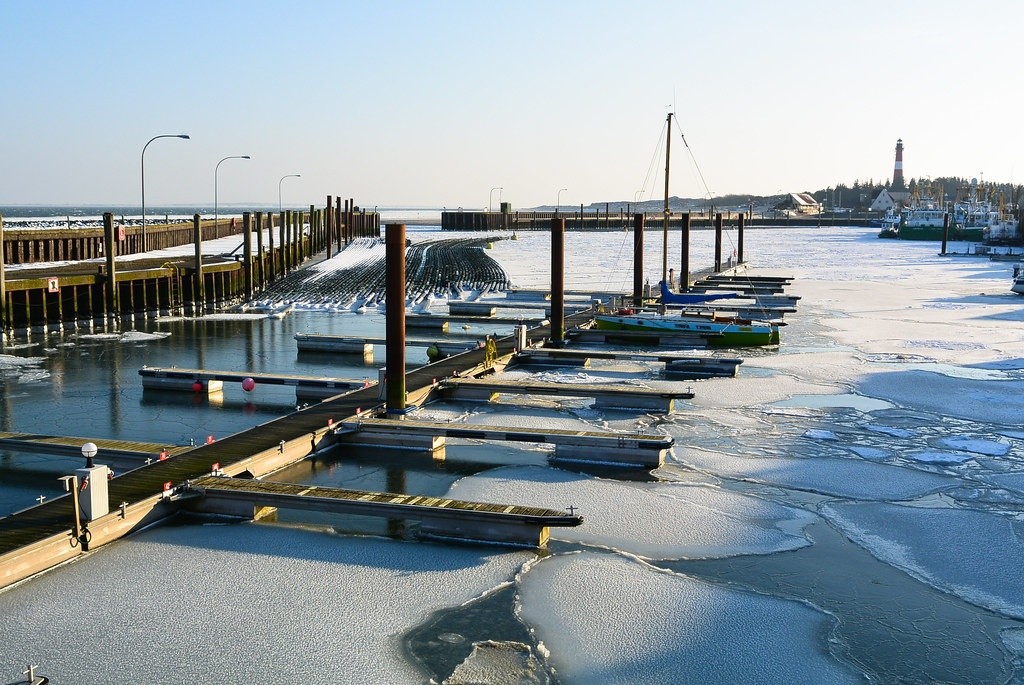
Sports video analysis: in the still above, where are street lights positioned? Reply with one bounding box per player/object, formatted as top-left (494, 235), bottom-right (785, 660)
top-left (490, 187), bottom-right (503, 211)
top-left (141, 134), bottom-right (190, 252)
top-left (558, 189), bottom-right (567, 211)
top-left (279, 174), bottom-right (300, 214)
top-left (214, 155), bottom-right (250, 239)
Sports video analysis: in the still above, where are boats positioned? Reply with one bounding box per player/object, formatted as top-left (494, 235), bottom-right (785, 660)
top-left (594, 312), bottom-right (780, 347)
